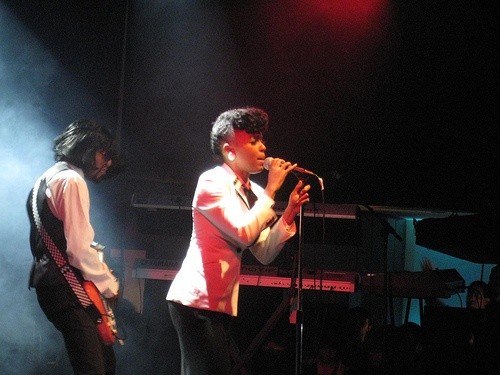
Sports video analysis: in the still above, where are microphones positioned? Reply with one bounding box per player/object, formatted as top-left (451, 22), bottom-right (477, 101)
top-left (263, 156), bottom-right (318, 177)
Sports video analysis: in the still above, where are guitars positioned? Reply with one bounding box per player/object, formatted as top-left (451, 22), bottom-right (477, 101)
top-left (83, 281), bottom-right (118, 346)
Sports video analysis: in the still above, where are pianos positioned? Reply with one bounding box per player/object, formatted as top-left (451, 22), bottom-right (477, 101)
top-left (133, 256), bottom-right (357, 294)
top-left (128, 190), bottom-right (357, 220)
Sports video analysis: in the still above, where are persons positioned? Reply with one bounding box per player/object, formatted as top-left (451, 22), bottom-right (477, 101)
top-left (26, 119), bottom-right (121, 375)
top-left (166, 107), bottom-right (310, 375)
top-left (306, 267), bottom-right (500, 375)
